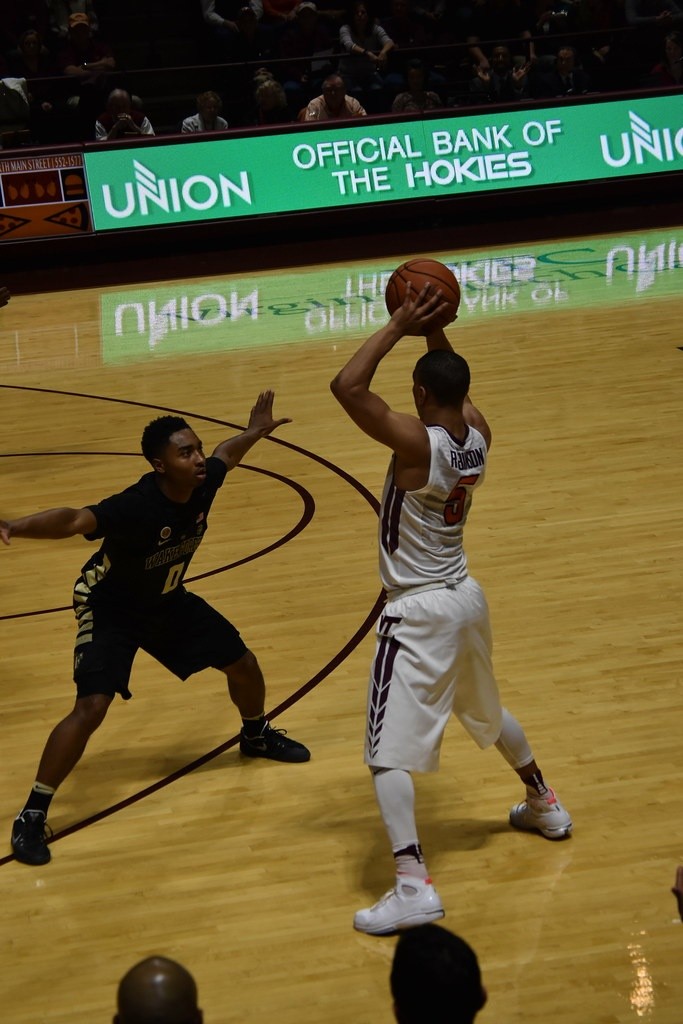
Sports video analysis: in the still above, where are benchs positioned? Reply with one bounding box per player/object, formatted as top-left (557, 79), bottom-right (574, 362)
top-left (1, 0), bottom-right (683, 137)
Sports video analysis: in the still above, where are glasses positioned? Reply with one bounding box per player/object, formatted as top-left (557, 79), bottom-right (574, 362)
top-left (24, 41), bottom-right (37, 45)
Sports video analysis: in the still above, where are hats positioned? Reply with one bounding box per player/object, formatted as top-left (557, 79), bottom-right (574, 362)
top-left (240, 7), bottom-right (257, 17)
top-left (296, 2), bottom-right (316, 14)
top-left (68, 12), bottom-right (90, 27)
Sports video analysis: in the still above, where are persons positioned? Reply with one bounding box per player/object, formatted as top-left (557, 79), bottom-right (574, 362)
top-left (0, 0), bottom-right (683, 146)
top-left (0, 389), bottom-right (309, 867)
top-left (113, 955), bottom-right (204, 1024)
top-left (330, 281), bottom-right (572, 933)
top-left (389, 923), bottom-right (487, 1024)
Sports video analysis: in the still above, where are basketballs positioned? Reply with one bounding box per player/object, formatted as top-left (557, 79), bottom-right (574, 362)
top-left (384, 258), bottom-right (461, 334)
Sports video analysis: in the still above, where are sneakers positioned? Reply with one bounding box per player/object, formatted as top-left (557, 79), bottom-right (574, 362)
top-left (12, 810), bottom-right (54, 865)
top-left (354, 879), bottom-right (445, 933)
top-left (510, 787), bottom-right (573, 837)
top-left (240, 720), bottom-right (310, 762)
